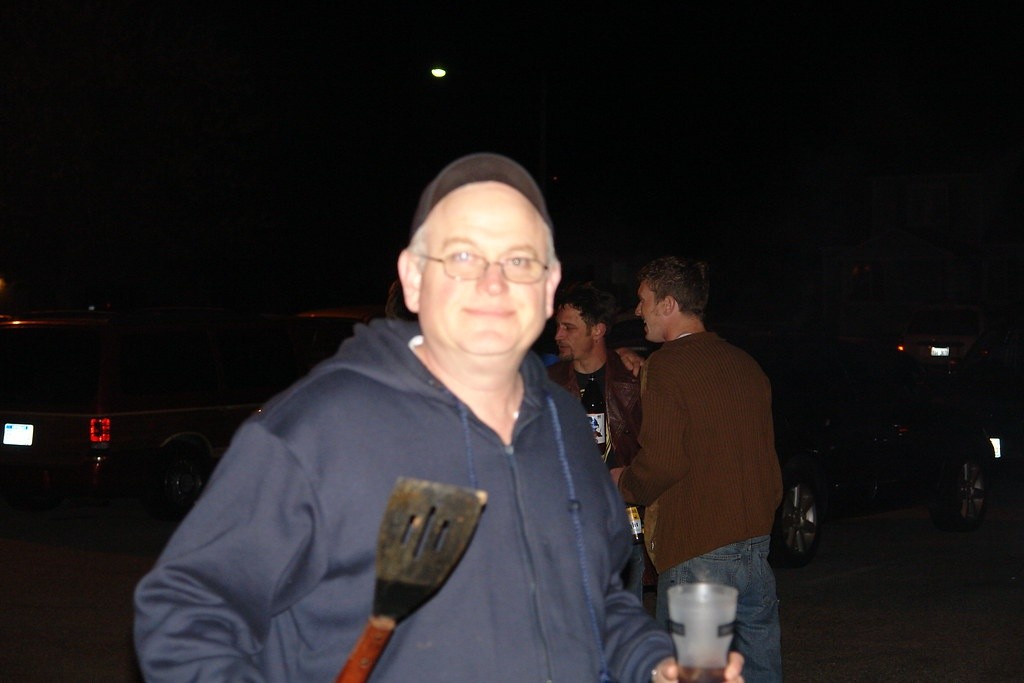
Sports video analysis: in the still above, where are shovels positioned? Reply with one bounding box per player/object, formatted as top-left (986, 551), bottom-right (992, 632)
top-left (334, 474), bottom-right (488, 683)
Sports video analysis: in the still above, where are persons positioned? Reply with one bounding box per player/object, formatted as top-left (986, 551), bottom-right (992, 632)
top-left (541, 254), bottom-right (784, 683)
top-left (133, 151), bottom-right (746, 683)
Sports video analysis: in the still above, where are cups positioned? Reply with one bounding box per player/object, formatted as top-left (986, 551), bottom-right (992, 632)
top-left (667, 583), bottom-right (738, 683)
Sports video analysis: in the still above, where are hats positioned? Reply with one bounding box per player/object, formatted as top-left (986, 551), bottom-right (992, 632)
top-left (410, 152), bottom-right (555, 246)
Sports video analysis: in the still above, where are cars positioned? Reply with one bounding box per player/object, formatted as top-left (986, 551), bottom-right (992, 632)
top-left (0, 306), bottom-right (1023, 569)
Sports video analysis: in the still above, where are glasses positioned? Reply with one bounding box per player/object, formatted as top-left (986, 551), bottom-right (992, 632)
top-left (422, 249), bottom-right (550, 284)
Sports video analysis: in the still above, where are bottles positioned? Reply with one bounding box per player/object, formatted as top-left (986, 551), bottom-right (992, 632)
top-left (624, 501), bottom-right (645, 545)
top-left (582, 374), bottom-right (606, 455)
